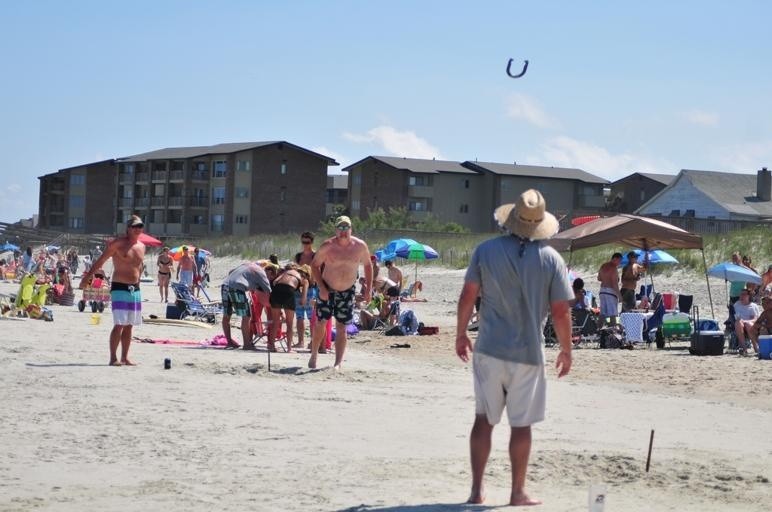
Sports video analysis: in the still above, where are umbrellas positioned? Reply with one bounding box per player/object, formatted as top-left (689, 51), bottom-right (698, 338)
top-left (707, 259), bottom-right (762, 305)
top-left (396, 243), bottom-right (438, 298)
top-left (375, 238), bottom-right (419, 265)
top-left (619, 248), bottom-right (679, 293)
top-left (174, 245), bottom-right (211, 261)
top-left (168, 246), bottom-right (181, 256)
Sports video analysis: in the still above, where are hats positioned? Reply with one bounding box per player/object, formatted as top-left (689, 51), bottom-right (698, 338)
top-left (334, 216), bottom-right (351, 227)
top-left (493, 188), bottom-right (560, 242)
top-left (127, 214), bottom-right (144, 225)
top-left (183, 245), bottom-right (188, 251)
top-left (297, 264), bottom-right (311, 275)
top-left (371, 255), bottom-right (376, 261)
top-left (626, 250), bottom-right (641, 258)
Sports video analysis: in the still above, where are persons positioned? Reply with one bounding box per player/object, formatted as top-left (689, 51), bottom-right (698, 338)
top-left (597, 253), bottom-right (623, 323)
top-left (751, 297), bottom-right (772, 336)
top-left (79, 215), bottom-right (146, 365)
top-left (191, 248), bottom-right (206, 298)
top-left (200, 248), bottom-right (210, 288)
top-left (0, 240), bottom-right (78, 306)
top-left (401, 281), bottom-right (422, 297)
top-left (569, 278), bottom-right (586, 310)
top-left (756, 265), bottom-right (772, 304)
top-left (157, 247), bottom-right (174, 303)
top-left (743, 256), bottom-right (758, 301)
top-left (372, 277), bottom-right (400, 301)
top-left (93, 245), bottom-right (103, 257)
top-left (308, 215), bottom-right (373, 371)
top-left (267, 264), bottom-right (311, 354)
top-left (360, 299), bottom-right (391, 329)
top-left (732, 289), bottom-right (760, 353)
top-left (311, 252), bottom-right (327, 353)
top-left (222, 263), bottom-right (279, 349)
top-left (294, 232), bottom-right (319, 349)
top-left (476, 289), bottom-right (481, 320)
top-left (455, 188), bottom-right (575, 508)
top-left (385, 261), bottom-right (402, 324)
top-left (370, 255), bottom-right (379, 279)
top-left (729, 251), bottom-right (747, 330)
top-left (355, 278), bottom-right (368, 308)
top-left (251, 264), bottom-right (296, 352)
top-left (176, 246), bottom-right (197, 296)
top-left (620, 251), bottom-right (647, 312)
top-left (269, 253), bottom-right (279, 264)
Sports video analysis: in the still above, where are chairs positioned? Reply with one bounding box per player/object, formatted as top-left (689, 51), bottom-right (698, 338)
top-left (248, 299), bottom-right (293, 353)
top-left (170, 282), bottom-right (224, 323)
top-left (371, 300), bottom-right (400, 331)
top-left (571, 310), bottom-right (593, 349)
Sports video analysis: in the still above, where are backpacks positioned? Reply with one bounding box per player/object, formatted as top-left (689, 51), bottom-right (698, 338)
top-left (600, 323), bottom-right (633, 350)
top-left (399, 310), bottom-right (418, 335)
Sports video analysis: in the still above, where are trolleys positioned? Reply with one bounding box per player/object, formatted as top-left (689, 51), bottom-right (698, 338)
top-left (655, 311), bottom-right (699, 350)
top-left (78, 278), bottom-right (111, 313)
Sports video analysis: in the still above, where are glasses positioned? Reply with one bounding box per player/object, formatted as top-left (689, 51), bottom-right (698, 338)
top-left (335, 226), bottom-right (351, 230)
top-left (302, 241), bottom-right (311, 244)
top-left (132, 225), bottom-right (143, 229)
top-left (388, 265), bottom-right (391, 269)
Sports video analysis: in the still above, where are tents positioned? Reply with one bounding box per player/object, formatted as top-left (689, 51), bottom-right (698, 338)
top-left (547, 213), bottom-right (715, 349)
